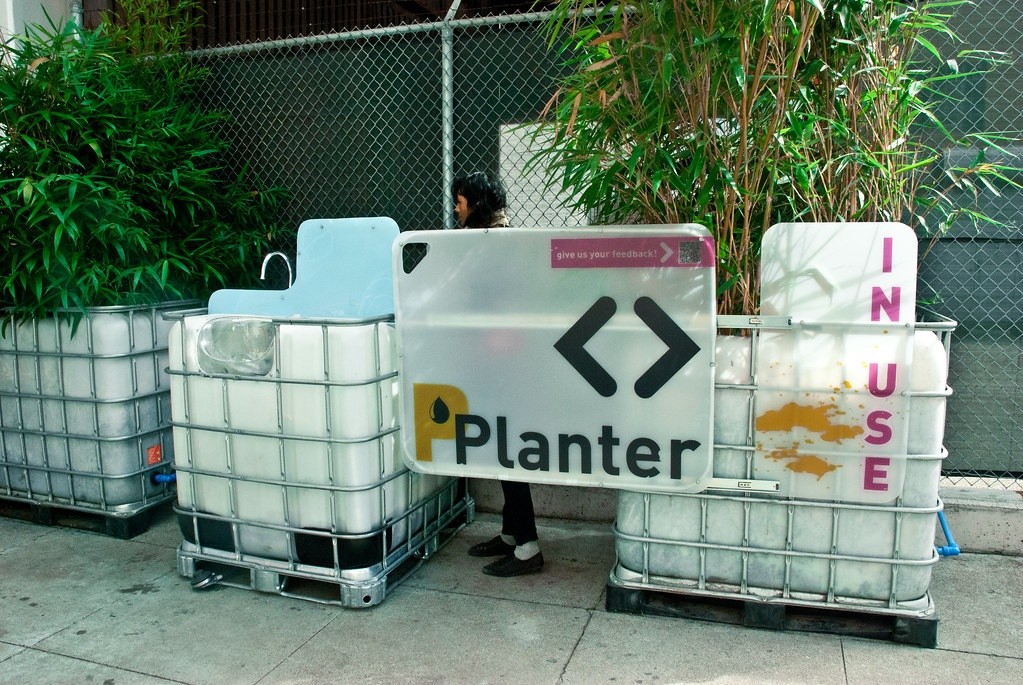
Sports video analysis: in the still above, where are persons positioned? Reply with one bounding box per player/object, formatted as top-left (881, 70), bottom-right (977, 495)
top-left (450, 173), bottom-right (545, 578)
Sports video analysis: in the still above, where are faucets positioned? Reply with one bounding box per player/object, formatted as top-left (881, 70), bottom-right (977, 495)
top-left (261, 250), bottom-right (294, 291)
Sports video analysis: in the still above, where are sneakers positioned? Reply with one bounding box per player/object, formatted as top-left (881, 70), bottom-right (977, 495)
top-left (482, 551), bottom-right (543, 577)
top-left (468, 535), bottom-right (515, 557)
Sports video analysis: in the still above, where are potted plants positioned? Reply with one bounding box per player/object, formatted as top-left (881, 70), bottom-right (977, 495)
top-left (541, 0), bottom-right (1023, 648)
top-left (0, 0), bottom-right (302, 542)
